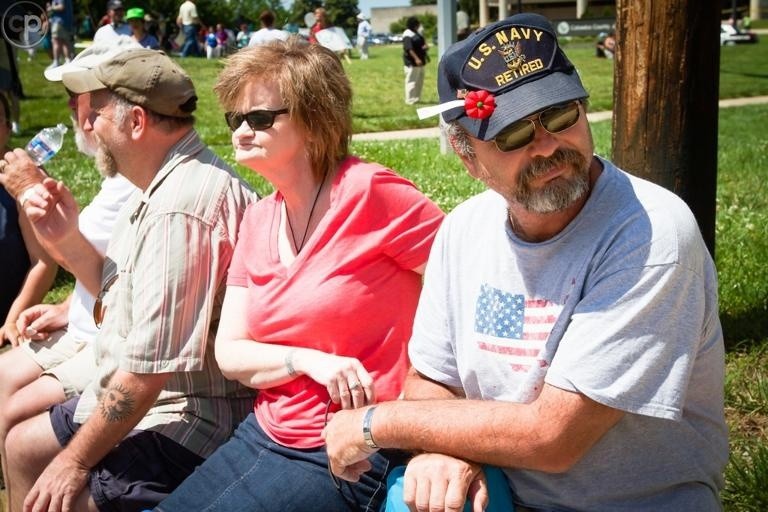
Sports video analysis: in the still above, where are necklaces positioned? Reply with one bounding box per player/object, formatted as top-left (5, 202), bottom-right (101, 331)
top-left (284, 166), bottom-right (328, 255)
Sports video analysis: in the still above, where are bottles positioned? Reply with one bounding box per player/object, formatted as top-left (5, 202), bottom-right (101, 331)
top-left (24, 123), bottom-right (70, 167)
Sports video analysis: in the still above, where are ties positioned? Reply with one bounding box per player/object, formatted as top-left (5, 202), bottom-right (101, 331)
top-left (42, 33), bottom-right (148, 86)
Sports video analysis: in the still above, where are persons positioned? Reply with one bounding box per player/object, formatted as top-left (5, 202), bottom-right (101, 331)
top-left (596, 31), bottom-right (615, 57)
top-left (474, 277), bottom-right (575, 372)
top-left (3, 47), bottom-right (261, 512)
top-left (0, 95), bottom-right (59, 348)
top-left (0, 35), bottom-right (149, 455)
top-left (141, 40), bottom-right (447, 512)
top-left (0, 36), bottom-right (26, 133)
top-left (402, 17), bottom-right (431, 105)
top-left (40, 1), bottom-right (371, 70)
top-left (99, 382), bottom-right (136, 423)
top-left (321, 12), bottom-right (730, 512)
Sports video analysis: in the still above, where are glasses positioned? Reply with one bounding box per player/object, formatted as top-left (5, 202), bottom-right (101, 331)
top-left (221, 108), bottom-right (291, 133)
top-left (455, 101), bottom-right (581, 154)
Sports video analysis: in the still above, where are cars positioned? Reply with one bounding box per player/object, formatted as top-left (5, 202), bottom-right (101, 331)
top-left (350, 34), bottom-right (403, 47)
top-left (720, 23), bottom-right (758, 48)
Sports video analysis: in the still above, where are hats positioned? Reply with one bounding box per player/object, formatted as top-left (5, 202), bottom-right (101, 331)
top-left (60, 47), bottom-right (201, 120)
top-left (106, 0), bottom-right (123, 11)
top-left (435, 12), bottom-right (591, 145)
top-left (355, 13), bottom-right (366, 21)
top-left (123, 7), bottom-right (145, 25)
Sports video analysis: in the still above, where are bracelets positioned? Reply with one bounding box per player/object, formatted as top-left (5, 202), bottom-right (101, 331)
top-left (285, 346), bottom-right (298, 379)
top-left (363, 406), bottom-right (377, 448)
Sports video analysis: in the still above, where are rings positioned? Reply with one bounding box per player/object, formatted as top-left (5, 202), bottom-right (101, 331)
top-left (1, 161), bottom-right (7, 172)
